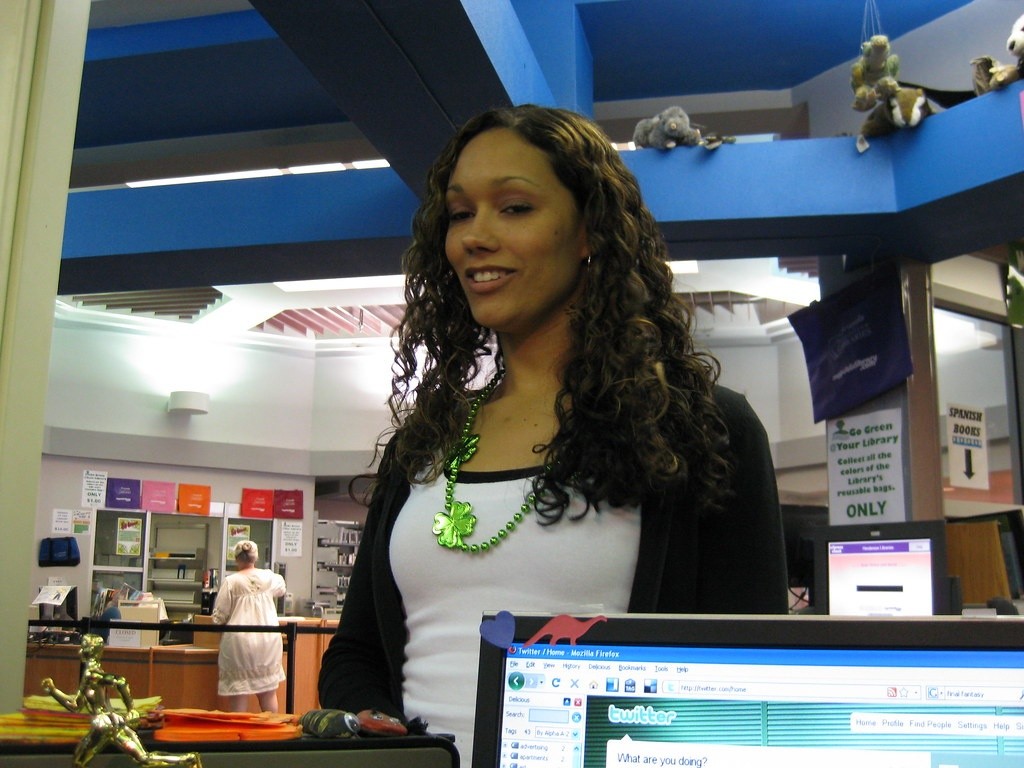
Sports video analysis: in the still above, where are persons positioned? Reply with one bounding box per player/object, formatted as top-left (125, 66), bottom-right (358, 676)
top-left (95, 601), bottom-right (124, 640)
top-left (41, 631), bottom-right (204, 768)
top-left (313, 102), bottom-right (795, 768)
top-left (211, 540), bottom-right (287, 713)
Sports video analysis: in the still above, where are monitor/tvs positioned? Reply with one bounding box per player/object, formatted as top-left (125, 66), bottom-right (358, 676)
top-left (812, 519), bottom-right (950, 615)
top-left (38, 586), bottom-right (78, 620)
top-left (469, 610), bottom-right (1024, 768)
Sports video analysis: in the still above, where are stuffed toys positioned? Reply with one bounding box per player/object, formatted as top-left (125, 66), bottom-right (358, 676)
top-left (300, 706), bottom-right (409, 736)
top-left (849, 32), bottom-right (902, 113)
top-left (968, 15), bottom-right (1024, 97)
top-left (855, 85), bottom-right (947, 154)
top-left (631, 105), bottom-right (702, 154)
top-left (704, 131), bottom-right (736, 152)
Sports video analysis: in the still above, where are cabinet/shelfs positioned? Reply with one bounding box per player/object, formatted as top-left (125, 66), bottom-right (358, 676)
top-left (322, 540), bottom-right (360, 589)
top-left (312, 511), bottom-right (337, 608)
top-left (147, 548), bottom-right (207, 611)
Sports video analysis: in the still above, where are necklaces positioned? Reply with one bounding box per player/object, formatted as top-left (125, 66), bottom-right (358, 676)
top-left (430, 368), bottom-right (575, 557)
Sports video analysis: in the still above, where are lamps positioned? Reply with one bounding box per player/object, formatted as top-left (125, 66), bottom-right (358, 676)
top-left (167, 391), bottom-right (209, 415)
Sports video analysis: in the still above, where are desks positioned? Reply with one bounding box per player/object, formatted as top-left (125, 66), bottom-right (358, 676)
top-left (0, 706), bottom-right (460, 768)
